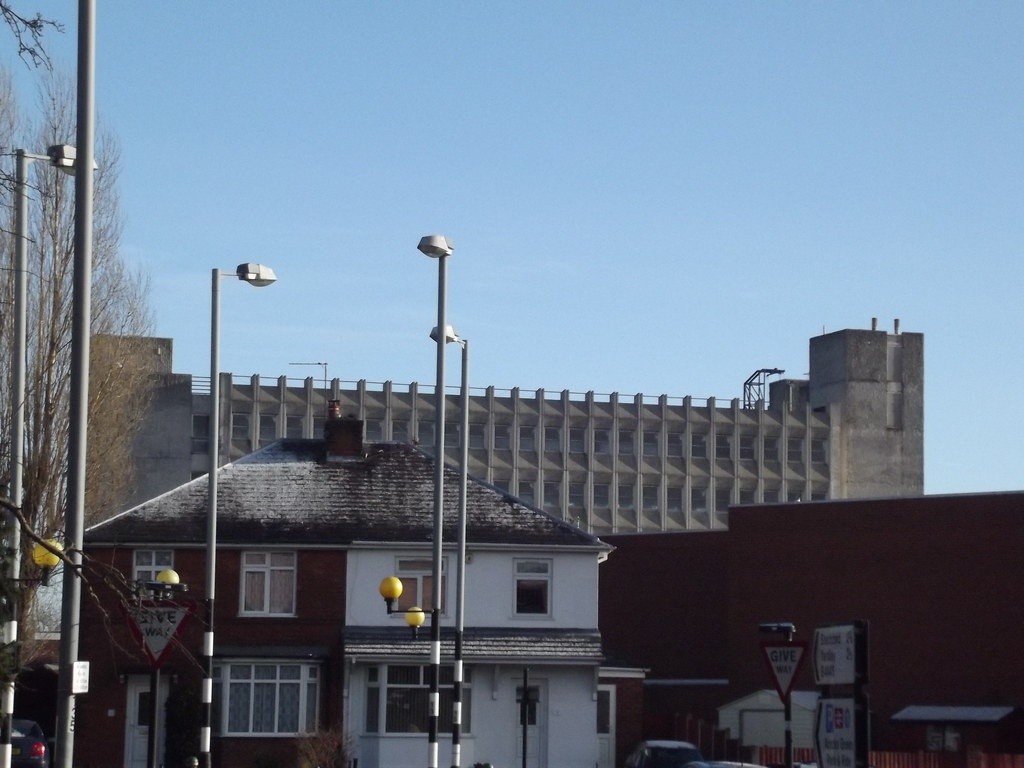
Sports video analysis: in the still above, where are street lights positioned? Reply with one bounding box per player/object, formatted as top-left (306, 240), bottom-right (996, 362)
top-left (147, 264), bottom-right (278, 766)
top-left (0, 144), bottom-right (98, 768)
top-left (380, 236), bottom-right (451, 767)
top-left (407, 325), bottom-right (468, 766)
top-left (758, 622), bottom-right (797, 768)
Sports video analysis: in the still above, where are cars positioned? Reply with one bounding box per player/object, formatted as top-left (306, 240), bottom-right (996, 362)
top-left (12, 719), bottom-right (51, 768)
top-left (626, 740), bottom-right (702, 768)
